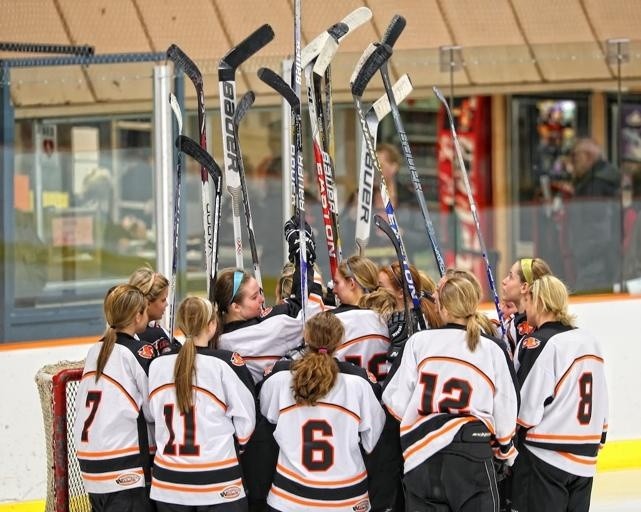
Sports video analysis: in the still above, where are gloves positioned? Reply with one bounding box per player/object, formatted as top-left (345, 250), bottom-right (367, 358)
top-left (495, 446), bottom-right (519, 467)
top-left (387, 312), bottom-right (413, 362)
top-left (284, 216), bottom-right (316, 259)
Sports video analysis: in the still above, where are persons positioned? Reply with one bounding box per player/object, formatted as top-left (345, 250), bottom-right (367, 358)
top-left (74, 141), bottom-right (433, 293)
top-left (563, 140), bottom-right (623, 289)
top-left (537, 106), bottom-right (576, 175)
top-left (259, 310), bottom-right (387, 510)
top-left (382, 278), bottom-right (518, 511)
top-left (513, 275), bottom-right (610, 510)
top-left (77, 220), bottom-right (612, 384)
top-left (79, 284), bottom-right (157, 510)
top-left (149, 295), bottom-right (259, 510)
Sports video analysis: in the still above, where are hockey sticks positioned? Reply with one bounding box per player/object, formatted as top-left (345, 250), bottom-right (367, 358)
top-left (153, 4), bottom-right (515, 369)
